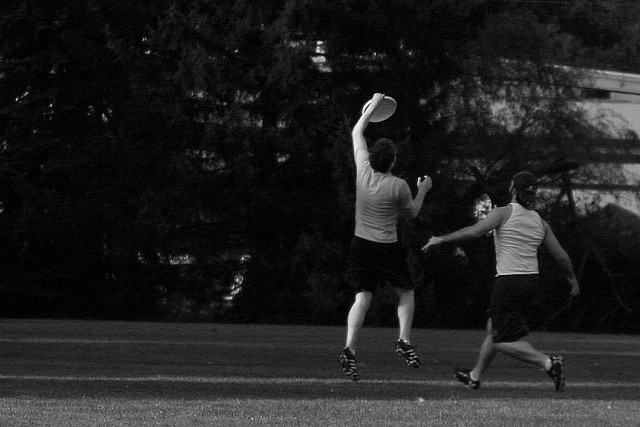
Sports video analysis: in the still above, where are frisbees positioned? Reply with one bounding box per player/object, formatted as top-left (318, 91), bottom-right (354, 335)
top-left (362, 97), bottom-right (397, 124)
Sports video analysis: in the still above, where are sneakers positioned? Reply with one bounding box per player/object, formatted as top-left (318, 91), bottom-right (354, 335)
top-left (544, 355), bottom-right (566, 391)
top-left (453, 367), bottom-right (480, 390)
top-left (338, 347), bottom-right (360, 381)
top-left (396, 339), bottom-right (422, 368)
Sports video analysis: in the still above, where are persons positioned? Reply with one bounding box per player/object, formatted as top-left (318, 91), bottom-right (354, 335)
top-left (421, 173), bottom-right (579, 392)
top-left (340, 90), bottom-right (432, 381)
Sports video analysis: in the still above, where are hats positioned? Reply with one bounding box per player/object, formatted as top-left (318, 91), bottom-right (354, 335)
top-left (513, 171), bottom-right (537, 202)
